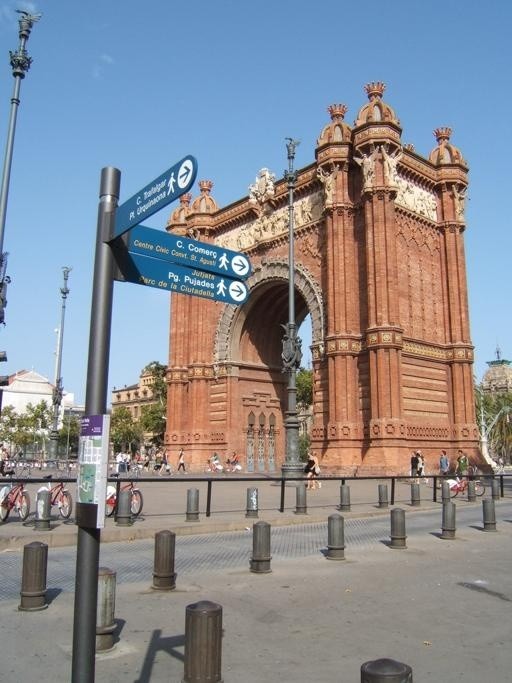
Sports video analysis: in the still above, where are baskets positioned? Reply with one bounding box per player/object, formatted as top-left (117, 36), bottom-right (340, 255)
top-left (469, 472), bottom-right (481, 480)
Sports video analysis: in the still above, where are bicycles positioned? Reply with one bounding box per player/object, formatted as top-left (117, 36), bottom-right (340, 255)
top-left (442, 466), bottom-right (485, 498)
top-left (0, 466), bottom-right (30, 520)
top-left (204, 459), bottom-right (223, 473)
top-left (36, 467), bottom-right (72, 518)
top-left (224, 459), bottom-right (243, 474)
top-left (106, 461), bottom-right (144, 518)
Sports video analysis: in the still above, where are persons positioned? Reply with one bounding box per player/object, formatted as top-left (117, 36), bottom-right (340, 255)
top-left (227, 452), bottom-right (238, 472)
top-left (409, 450), bottom-right (428, 484)
top-left (305, 451), bottom-right (317, 489)
top-left (210, 452), bottom-right (220, 470)
top-left (114, 445), bottom-right (188, 475)
top-left (437, 449), bottom-right (450, 488)
top-left (0, 444), bottom-right (10, 477)
top-left (454, 449), bottom-right (469, 480)
top-left (311, 452), bottom-right (321, 488)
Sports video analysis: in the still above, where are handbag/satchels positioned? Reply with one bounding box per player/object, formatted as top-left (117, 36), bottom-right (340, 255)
top-left (165, 464), bottom-right (170, 470)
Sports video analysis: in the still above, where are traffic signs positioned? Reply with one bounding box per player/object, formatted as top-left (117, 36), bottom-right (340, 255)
top-left (116, 224), bottom-right (252, 303)
top-left (112, 154), bottom-right (196, 240)
